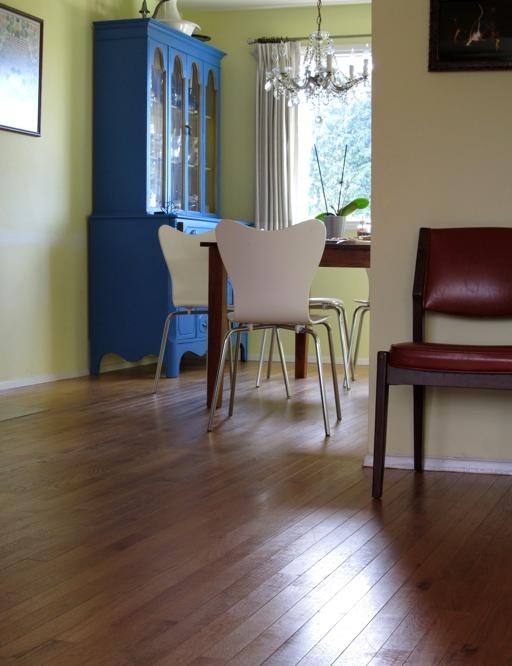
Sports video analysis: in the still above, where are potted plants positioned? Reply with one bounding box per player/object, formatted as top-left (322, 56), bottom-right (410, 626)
top-left (314, 197), bottom-right (368, 238)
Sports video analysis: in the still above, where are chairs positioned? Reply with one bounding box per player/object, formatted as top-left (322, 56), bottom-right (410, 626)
top-left (207, 219), bottom-right (342, 436)
top-left (369, 224), bottom-right (512, 498)
top-left (152, 224), bottom-right (234, 394)
top-left (256, 297), bottom-right (369, 389)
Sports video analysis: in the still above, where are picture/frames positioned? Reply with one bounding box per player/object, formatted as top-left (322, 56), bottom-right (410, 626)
top-left (426, 0), bottom-right (511, 73)
top-left (0, 1), bottom-right (44, 136)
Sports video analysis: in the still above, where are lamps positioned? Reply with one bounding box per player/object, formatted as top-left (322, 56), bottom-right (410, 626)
top-left (264, 0), bottom-right (371, 105)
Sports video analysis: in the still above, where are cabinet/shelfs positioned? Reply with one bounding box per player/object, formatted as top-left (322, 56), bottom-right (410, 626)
top-left (94, 18), bottom-right (168, 219)
top-left (199, 40), bottom-right (227, 219)
top-left (167, 26), bottom-right (204, 220)
top-left (200, 238), bottom-right (370, 408)
top-left (87, 215), bottom-right (250, 378)
